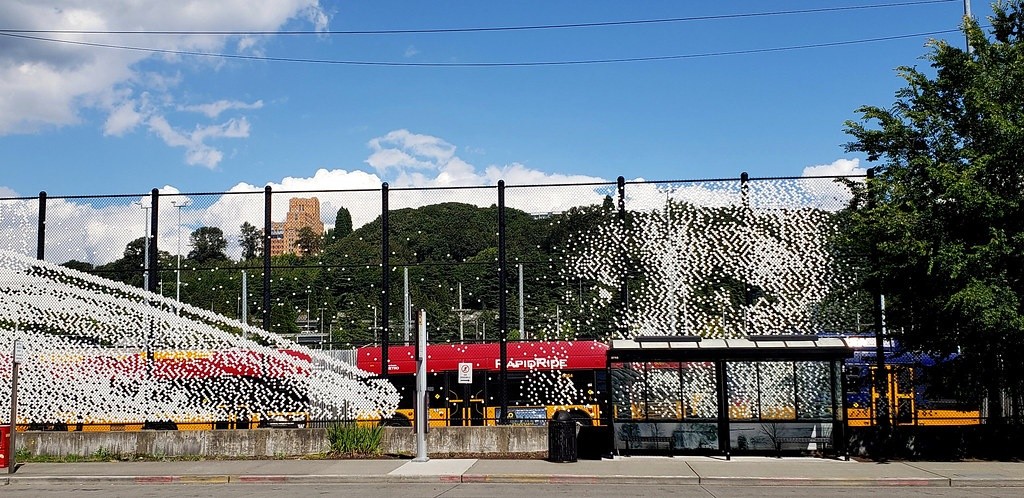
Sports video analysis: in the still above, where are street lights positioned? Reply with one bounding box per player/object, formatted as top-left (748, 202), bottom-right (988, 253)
top-left (133, 200), bottom-right (154, 295)
top-left (171, 200), bottom-right (191, 302)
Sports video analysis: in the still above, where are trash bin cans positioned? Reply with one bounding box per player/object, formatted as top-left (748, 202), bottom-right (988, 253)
top-left (548, 410), bottom-right (577, 463)
top-left (0, 425), bottom-right (10, 468)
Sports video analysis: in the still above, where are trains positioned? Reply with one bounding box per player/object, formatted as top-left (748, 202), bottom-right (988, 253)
top-left (1, 337), bottom-right (989, 437)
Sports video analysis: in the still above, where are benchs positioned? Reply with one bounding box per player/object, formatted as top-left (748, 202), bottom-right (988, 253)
top-left (774, 436), bottom-right (831, 459)
top-left (620, 436), bottom-right (676, 458)
